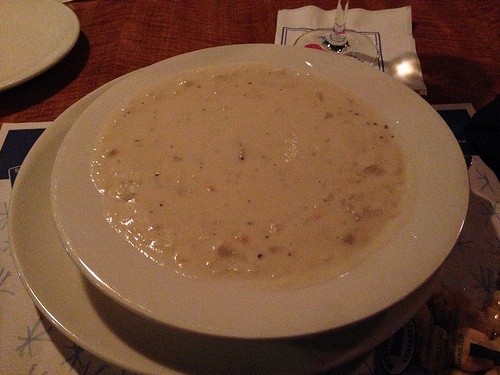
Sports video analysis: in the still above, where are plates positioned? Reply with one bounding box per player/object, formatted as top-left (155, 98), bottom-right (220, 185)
top-left (0, 0), bottom-right (81, 94)
top-left (51, 46), bottom-right (469, 337)
top-left (5, 70), bottom-right (446, 375)
